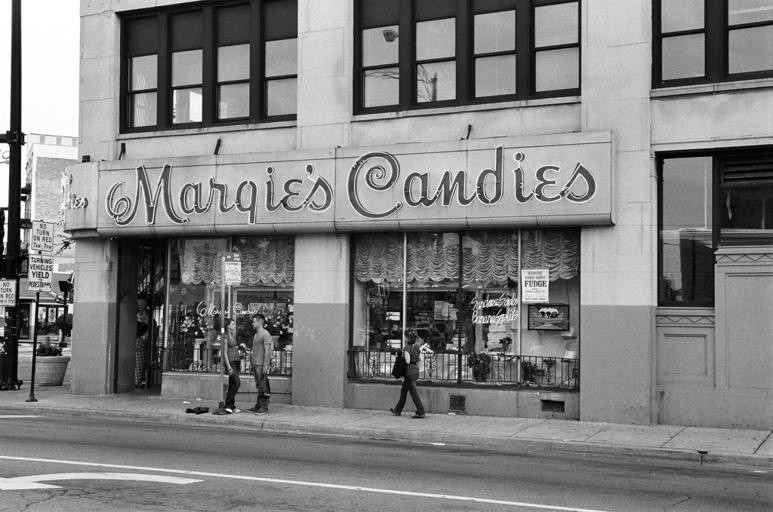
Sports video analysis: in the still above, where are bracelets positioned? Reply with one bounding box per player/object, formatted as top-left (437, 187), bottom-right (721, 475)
top-left (226, 367), bottom-right (232, 372)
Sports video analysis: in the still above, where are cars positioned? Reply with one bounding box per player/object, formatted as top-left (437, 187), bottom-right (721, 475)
top-left (236, 319), bottom-right (292, 351)
top-left (382, 328), bottom-right (447, 355)
top-left (169, 332), bottom-right (222, 369)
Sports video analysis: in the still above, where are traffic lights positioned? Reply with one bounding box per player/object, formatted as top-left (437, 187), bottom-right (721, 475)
top-left (16, 256), bottom-right (28, 277)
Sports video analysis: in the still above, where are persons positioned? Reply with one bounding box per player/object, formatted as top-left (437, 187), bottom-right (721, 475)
top-left (222, 318), bottom-right (246, 414)
top-left (389, 329), bottom-right (425, 419)
top-left (135, 307), bottom-right (150, 390)
top-left (245, 314), bottom-right (271, 416)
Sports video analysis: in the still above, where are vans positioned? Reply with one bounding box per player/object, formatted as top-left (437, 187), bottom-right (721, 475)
top-left (411, 322), bottom-right (450, 343)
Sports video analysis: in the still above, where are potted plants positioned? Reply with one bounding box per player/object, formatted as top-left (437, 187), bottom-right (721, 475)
top-left (30, 341), bottom-right (71, 387)
